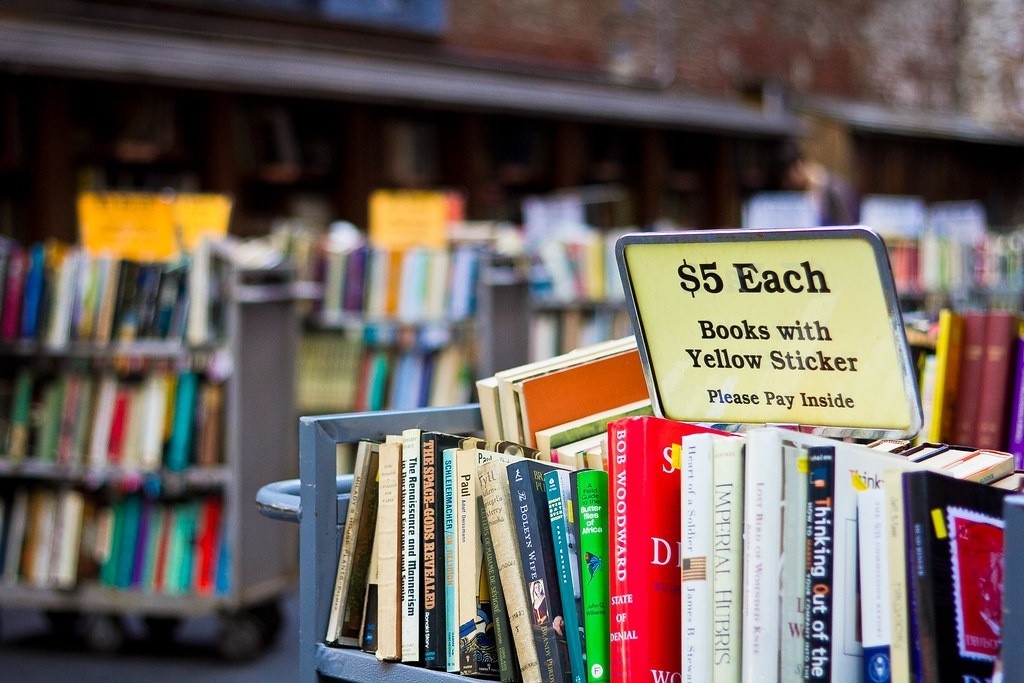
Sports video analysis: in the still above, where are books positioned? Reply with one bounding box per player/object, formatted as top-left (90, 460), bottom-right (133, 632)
top-left (269, 224), bottom-right (476, 324)
top-left (0, 489), bottom-right (224, 595)
top-left (1, 237), bottom-right (253, 349)
top-left (491, 192), bottom-right (638, 300)
top-left (294, 331), bottom-right (474, 476)
top-left (743, 198), bottom-right (1022, 307)
top-left (322, 308), bottom-right (1024, 683)
top-left (0, 365), bottom-right (226, 476)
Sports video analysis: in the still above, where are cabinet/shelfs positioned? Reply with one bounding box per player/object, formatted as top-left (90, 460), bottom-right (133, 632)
top-left (0, 270), bottom-right (539, 660)
